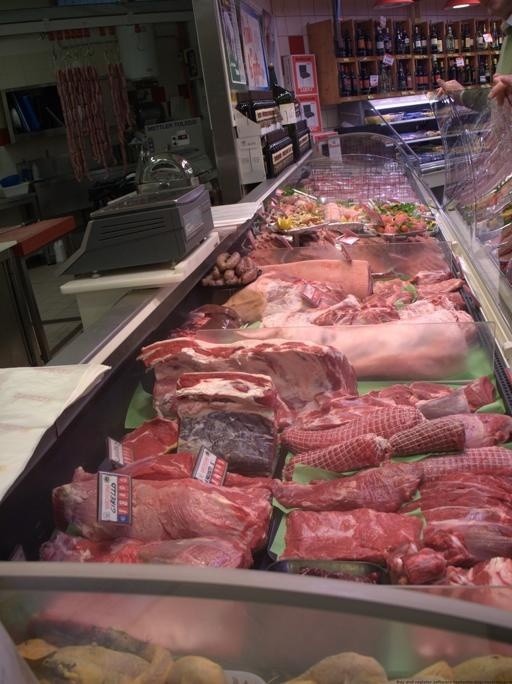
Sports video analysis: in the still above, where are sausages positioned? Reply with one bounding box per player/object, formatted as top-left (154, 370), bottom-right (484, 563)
top-left (200, 251), bottom-right (257, 287)
top-left (55, 61), bottom-right (133, 183)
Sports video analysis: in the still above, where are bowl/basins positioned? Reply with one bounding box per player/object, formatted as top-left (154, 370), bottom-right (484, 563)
top-left (0, 174), bottom-right (29, 199)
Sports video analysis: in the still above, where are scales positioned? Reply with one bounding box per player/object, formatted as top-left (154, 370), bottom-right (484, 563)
top-left (59, 184), bottom-right (215, 273)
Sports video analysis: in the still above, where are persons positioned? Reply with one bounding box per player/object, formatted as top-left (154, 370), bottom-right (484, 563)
top-left (430, 0), bottom-right (511, 278)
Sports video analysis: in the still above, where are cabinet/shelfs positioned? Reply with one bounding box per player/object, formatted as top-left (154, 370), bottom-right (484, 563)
top-left (308, 16), bottom-right (505, 105)
top-left (336, 89), bottom-right (492, 189)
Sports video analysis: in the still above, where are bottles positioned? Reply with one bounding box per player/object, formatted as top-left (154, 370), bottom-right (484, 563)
top-left (242, 11), bottom-right (263, 87)
top-left (236, 65), bottom-right (311, 177)
top-left (335, 21), bottom-right (505, 96)
